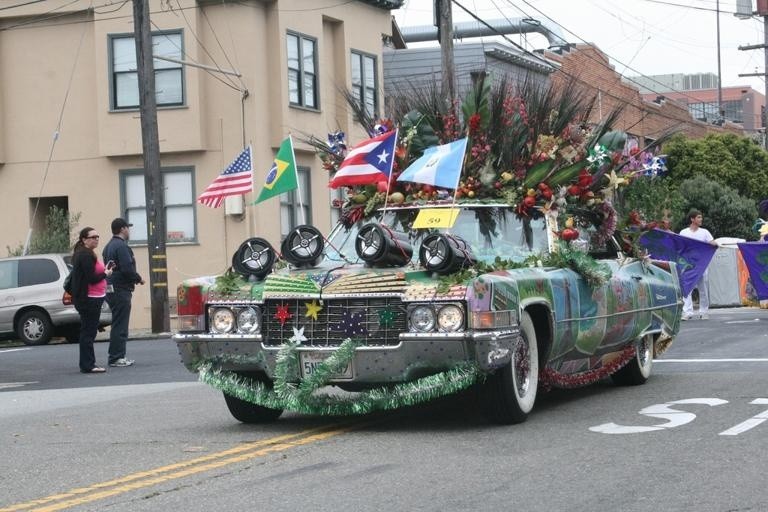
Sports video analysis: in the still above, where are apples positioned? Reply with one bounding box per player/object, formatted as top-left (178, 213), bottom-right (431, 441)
top-left (527, 158), bottom-right (534, 166)
top-left (524, 183), bottom-right (578, 208)
top-left (562, 229), bottom-right (579, 240)
top-left (455, 178), bottom-right (481, 197)
top-left (494, 180), bottom-right (502, 189)
top-left (387, 184), bottom-right (437, 202)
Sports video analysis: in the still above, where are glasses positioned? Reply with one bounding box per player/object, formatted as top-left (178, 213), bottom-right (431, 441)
top-left (84, 236), bottom-right (100, 239)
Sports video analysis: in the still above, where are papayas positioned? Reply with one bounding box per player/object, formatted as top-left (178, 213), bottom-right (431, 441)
top-left (352, 195), bottom-right (366, 204)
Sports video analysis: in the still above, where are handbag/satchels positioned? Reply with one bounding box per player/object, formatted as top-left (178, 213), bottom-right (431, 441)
top-left (63, 270), bottom-right (73, 295)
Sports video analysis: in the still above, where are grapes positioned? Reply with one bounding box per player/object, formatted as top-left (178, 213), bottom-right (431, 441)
top-left (364, 192), bottom-right (385, 216)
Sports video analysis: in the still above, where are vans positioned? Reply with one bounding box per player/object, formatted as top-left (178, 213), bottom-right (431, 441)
top-left (0, 252), bottom-right (112, 347)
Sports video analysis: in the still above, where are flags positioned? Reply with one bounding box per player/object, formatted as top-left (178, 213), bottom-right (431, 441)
top-left (250, 137), bottom-right (297, 205)
top-left (739, 242), bottom-right (768, 303)
top-left (631, 226), bottom-right (716, 298)
top-left (397, 137), bottom-right (468, 190)
top-left (331, 128), bottom-right (396, 190)
top-left (196, 147), bottom-right (253, 209)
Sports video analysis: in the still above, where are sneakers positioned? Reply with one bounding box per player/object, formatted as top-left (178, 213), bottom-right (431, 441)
top-left (681, 314), bottom-right (712, 321)
top-left (80, 366), bottom-right (106, 372)
top-left (108, 356), bottom-right (137, 367)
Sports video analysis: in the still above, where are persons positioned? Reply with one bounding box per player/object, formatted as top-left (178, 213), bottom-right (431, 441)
top-left (661, 220), bottom-right (671, 235)
top-left (678, 209), bottom-right (718, 321)
top-left (103, 218), bottom-right (145, 366)
top-left (71, 227), bottom-right (113, 371)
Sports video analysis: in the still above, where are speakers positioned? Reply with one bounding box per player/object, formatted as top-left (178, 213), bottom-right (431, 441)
top-left (418, 234), bottom-right (477, 273)
top-left (354, 223), bottom-right (412, 266)
top-left (231, 237), bottom-right (275, 282)
top-left (281, 224), bottom-right (324, 267)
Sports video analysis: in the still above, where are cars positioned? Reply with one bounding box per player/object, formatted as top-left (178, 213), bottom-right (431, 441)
top-left (172, 190), bottom-right (687, 427)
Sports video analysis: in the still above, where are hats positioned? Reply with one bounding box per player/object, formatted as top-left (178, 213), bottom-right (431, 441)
top-left (112, 218), bottom-right (133, 231)
